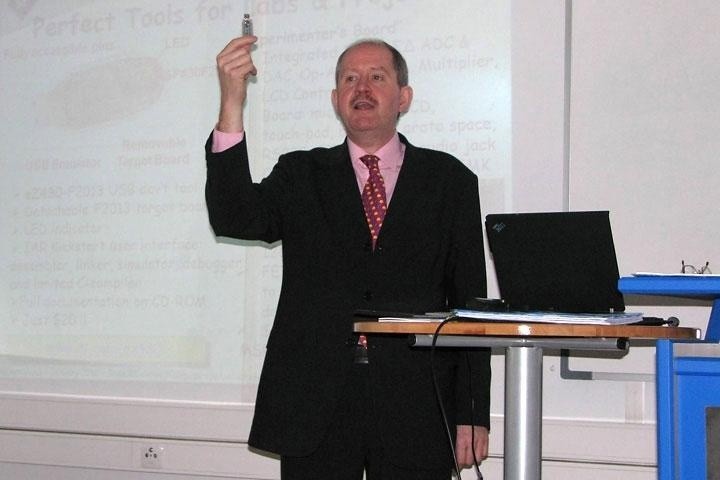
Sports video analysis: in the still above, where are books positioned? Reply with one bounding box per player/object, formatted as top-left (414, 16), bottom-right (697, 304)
top-left (455, 307), bottom-right (644, 326)
top-left (378, 307), bottom-right (457, 325)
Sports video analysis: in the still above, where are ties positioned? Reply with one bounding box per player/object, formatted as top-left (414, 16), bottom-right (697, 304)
top-left (358, 155), bottom-right (388, 348)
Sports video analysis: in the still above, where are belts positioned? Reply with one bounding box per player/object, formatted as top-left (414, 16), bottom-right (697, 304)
top-left (354, 346), bottom-right (369, 364)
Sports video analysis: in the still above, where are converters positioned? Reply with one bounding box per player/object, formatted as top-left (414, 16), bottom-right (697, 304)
top-left (451, 294), bottom-right (506, 313)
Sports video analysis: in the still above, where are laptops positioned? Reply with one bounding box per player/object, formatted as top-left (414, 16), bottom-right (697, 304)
top-left (485, 211), bottom-right (664, 326)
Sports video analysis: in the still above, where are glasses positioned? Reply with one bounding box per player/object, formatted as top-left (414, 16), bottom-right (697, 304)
top-left (681, 260), bottom-right (711, 274)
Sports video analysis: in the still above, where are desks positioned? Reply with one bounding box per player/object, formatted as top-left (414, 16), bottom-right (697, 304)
top-left (351, 317), bottom-right (699, 477)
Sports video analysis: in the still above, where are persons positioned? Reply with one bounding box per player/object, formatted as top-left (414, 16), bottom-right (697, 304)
top-left (202, 34), bottom-right (493, 479)
